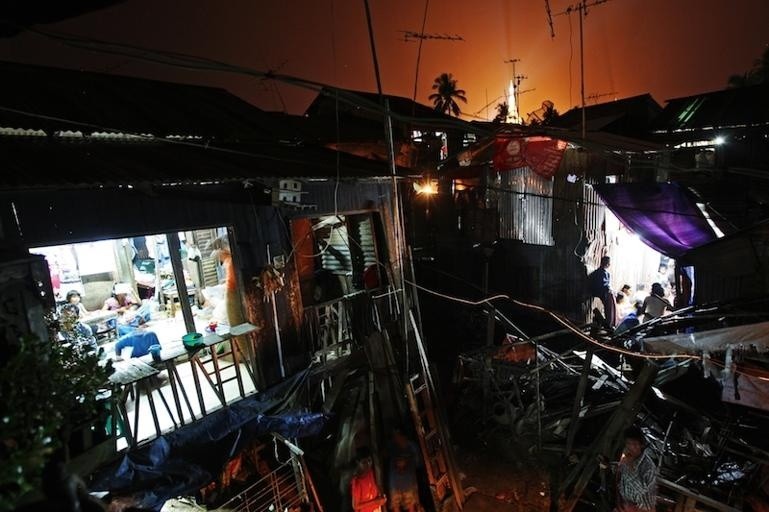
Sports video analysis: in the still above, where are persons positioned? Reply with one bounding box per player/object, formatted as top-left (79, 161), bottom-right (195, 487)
top-left (334, 410), bottom-right (424, 512)
top-left (596, 257), bottom-right (692, 339)
top-left (54, 282), bottom-right (166, 378)
top-left (614, 426), bottom-right (658, 512)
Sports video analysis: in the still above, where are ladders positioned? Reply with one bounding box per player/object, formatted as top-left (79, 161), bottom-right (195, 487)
top-left (406, 372), bottom-right (463, 512)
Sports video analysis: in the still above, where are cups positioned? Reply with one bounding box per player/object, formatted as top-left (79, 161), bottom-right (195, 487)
top-left (149, 344), bottom-right (161, 360)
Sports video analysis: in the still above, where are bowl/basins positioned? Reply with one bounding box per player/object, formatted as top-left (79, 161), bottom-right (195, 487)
top-left (182, 332), bottom-right (203, 350)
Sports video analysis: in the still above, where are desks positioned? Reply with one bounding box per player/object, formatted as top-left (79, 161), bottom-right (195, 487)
top-left (104, 356), bottom-right (160, 446)
